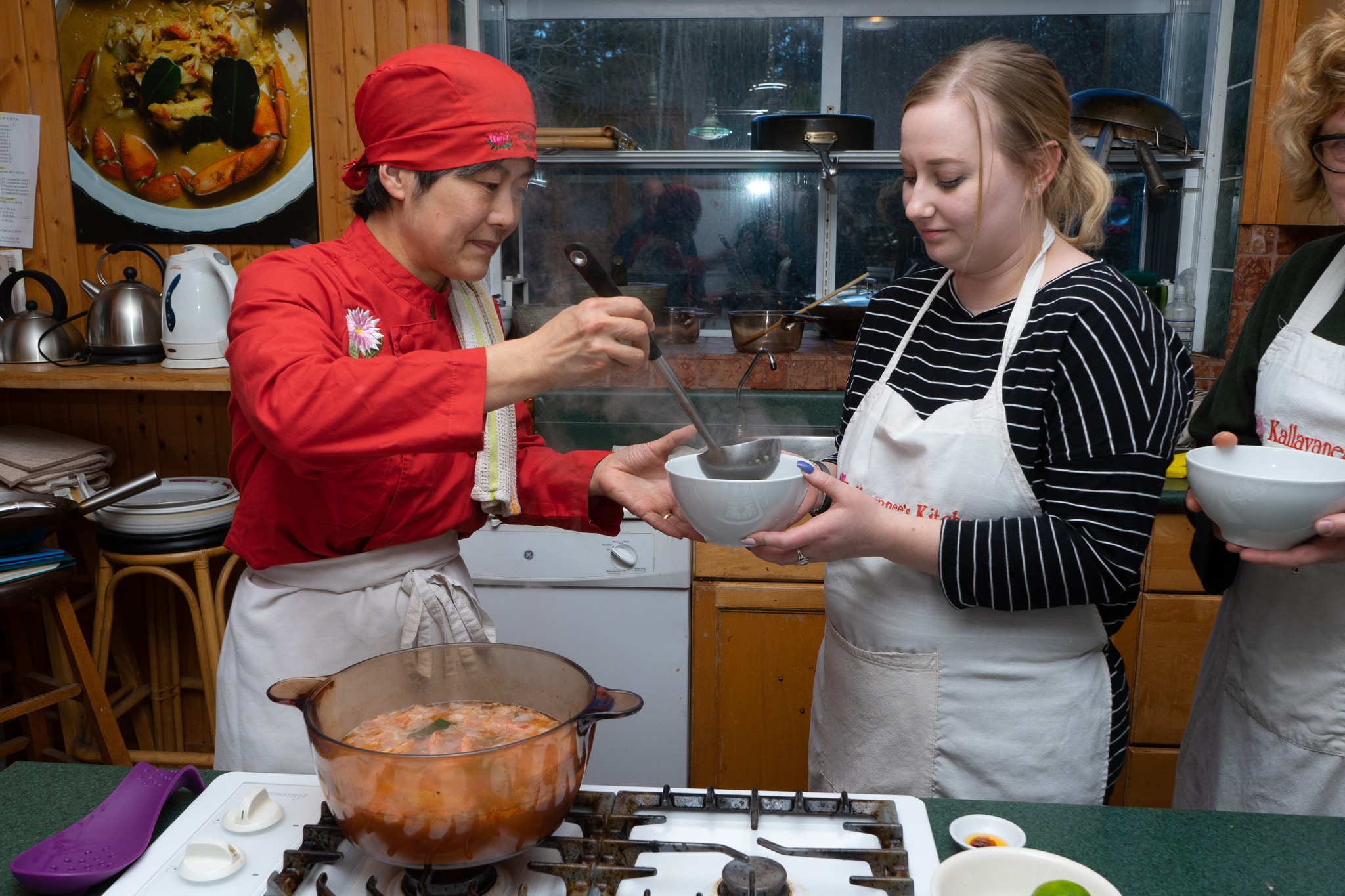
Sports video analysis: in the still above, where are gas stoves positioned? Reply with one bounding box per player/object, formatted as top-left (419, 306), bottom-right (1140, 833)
top-left (101, 771), bottom-right (938, 896)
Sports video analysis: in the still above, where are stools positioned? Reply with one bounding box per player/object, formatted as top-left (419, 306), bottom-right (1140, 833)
top-left (0, 547), bottom-right (133, 766)
top-left (86, 528), bottom-right (241, 770)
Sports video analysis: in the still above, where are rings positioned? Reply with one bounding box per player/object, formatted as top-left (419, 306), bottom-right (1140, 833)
top-left (797, 548), bottom-right (809, 566)
top-left (663, 514), bottom-right (674, 520)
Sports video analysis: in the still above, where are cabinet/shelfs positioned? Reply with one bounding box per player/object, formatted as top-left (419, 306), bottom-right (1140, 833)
top-left (688, 514), bottom-right (1226, 809)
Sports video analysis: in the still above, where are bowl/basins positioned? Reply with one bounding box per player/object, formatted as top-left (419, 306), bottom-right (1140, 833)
top-left (515, 303), bottom-right (571, 336)
top-left (573, 282), bottom-right (669, 313)
top-left (1186, 445), bottom-right (1345, 550)
top-left (664, 452), bottom-right (814, 547)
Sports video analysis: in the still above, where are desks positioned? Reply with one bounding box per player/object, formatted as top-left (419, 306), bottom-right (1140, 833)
top-left (0, 356), bottom-right (231, 392)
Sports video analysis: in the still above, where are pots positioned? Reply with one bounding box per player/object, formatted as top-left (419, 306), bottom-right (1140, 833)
top-left (799, 276), bottom-right (889, 341)
top-left (0, 470), bottom-right (161, 551)
top-left (751, 113), bottom-right (876, 175)
top-left (646, 307), bottom-right (714, 343)
top-left (1070, 87), bottom-right (1196, 195)
top-left (728, 311), bottom-right (826, 353)
top-left (267, 643), bottom-right (644, 868)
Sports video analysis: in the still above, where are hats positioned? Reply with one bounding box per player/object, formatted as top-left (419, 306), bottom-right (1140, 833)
top-left (341, 45), bottom-right (536, 192)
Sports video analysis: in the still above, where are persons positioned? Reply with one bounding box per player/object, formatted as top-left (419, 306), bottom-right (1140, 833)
top-left (728, 182), bottom-right (813, 310)
top-left (214, 42), bottom-right (707, 772)
top-left (739, 41), bottom-right (1197, 805)
top-left (610, 179), bottom-right (705, 299)
top-left (891, 175), bottom-right (941, 281)
top-left (627, 184), bottom-right (736, 306)
top-left (876, 185), bottom-right (912, 238)
top-left (835, 200), bottom-right (856, 287)
top-left (1176, 8), bottom-right (1345, 818)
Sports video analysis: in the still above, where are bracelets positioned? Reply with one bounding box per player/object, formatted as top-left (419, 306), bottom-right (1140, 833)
top-left (807, 460), bottom-right (826, 514)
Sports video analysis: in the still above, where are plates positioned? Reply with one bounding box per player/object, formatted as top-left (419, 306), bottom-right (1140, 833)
top-left (92, 476), bottom-right (240, 534)
top-left (928, 847), bottom-right (1122, 896)
top-left (949, 814), bottom-right (1027, 850)
top-left (53, 0), bottom-right (314, 232)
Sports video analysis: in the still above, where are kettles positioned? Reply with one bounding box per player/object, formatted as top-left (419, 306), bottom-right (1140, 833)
top-left (0, 267), bottom-right (87, 363)
top-left (160, 243), bottom-right (239, 369)
top-left (80, 243), bottom-right (166, 364)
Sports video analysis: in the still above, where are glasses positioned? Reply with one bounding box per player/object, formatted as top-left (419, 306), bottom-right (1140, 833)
top-left (1307, 125), bottom-right (1345, 174)
